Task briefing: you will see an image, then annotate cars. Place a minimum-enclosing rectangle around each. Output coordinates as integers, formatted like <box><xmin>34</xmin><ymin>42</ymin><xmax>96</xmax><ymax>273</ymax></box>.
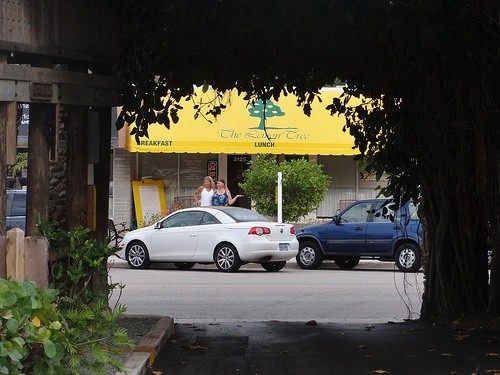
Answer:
<box><xmin>7</xmin><ymin>186</ymin><xmax>27</xmax><ymax>234</ymax></box>
<box><xmin>124</xmin><ymin>206</ymin><xmax>299</xmax><ymax>273</ymax></box>
<box><xmin>296</xmin><ymin>200</ymin><xmax>424</xmax><ymax>273</ymax></box>
